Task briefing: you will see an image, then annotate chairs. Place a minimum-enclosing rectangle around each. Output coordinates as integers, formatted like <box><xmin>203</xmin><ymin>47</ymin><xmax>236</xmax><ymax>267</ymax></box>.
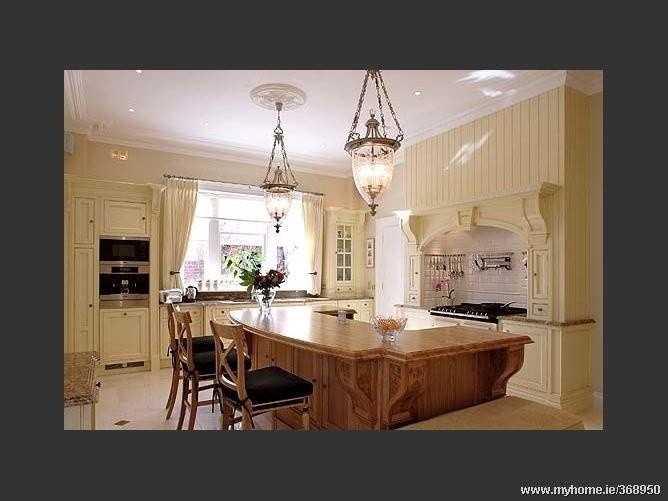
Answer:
<box><xmin>159</xmin><ymin>303</ymin><xmax>312</xmax><ymax>431</ymax></box>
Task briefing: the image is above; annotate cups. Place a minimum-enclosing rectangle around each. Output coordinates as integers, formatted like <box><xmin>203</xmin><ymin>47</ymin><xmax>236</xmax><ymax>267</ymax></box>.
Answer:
<box><xmin>338</xmin><ymin>311</ymin><xmax>346</xmax><ymax>323</ymax></box>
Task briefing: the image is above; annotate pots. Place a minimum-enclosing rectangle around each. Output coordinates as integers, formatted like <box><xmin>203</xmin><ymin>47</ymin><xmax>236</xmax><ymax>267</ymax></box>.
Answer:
<box><xmin>480</xmin><ymin>301</ymin><xmax>515</xmax><ymax>310</ymax></box>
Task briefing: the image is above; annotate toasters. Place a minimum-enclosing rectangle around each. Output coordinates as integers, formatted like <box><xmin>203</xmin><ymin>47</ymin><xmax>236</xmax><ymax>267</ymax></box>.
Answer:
<box><xmin>159</xmin><ymin>290</ymin><xmax>182</xmax><ymax>303</ymax></box>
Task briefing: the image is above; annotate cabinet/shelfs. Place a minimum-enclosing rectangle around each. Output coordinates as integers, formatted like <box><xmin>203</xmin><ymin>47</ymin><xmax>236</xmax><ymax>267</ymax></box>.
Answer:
<box><xmin>101</xmin><ymin>198</ymin><xmax>152</xmax><ymax>236</ymax></box>
<box><xmin>162</xmin><ymin>304</ymin><xmax>235</xmax><ymax>368</ymax></box>
<box><xmin>65</xmin><ymin>173</ymin><xmax>100</xmax><ymax>376</ymax></box>
<box><xmin>100</xmin><ymin>308</ymin><xmax>150</xmax><ymax>370</ymax></box>
<box><xmin>226</xmin><ymin>330</ymin><xmax>351</xmax><ymax>430</ymax></box>
<box><xmin>338</xmin><ymin>300</ymin><xmax>375</xmax><ymax>321</ymax></box>
<box><xmin>322</xmin><ymin>208</ymin><xmax>365</xmax><ymax>298</ymax></box>
<box><xmin>401</xmin><ymin>311</ymin><xmax>551</xmax><ymax>396</ymax></box>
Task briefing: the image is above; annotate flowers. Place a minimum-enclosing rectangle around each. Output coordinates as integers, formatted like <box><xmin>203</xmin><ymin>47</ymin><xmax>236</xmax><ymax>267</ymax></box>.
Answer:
<box><xmin>223</xmin><ymin>245</ymin><xmax>288</xmax><ymax>307</ymax></box>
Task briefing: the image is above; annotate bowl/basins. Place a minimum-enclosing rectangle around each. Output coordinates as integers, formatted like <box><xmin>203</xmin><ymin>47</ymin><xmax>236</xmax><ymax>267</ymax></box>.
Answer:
<box><xmin>370</xmin><ymin>316</ymin><xmax>409</xmax><ymax>343</ymax></box>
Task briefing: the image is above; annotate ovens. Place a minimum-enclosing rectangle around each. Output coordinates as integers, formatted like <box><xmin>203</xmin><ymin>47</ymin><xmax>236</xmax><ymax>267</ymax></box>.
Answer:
<box><xmin>99</xmin><ymin>265</ymin><xmax>151</xmax><ymax>364</ymax></box>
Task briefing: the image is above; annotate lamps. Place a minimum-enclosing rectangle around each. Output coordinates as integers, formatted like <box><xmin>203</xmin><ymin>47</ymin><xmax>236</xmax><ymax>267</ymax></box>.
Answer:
<box><xmin>249</xmin><ymin>83</ymin><xmax>310</xmax><ymax>236</ymax></box>
<box><xmin>343</xmin><ymin>69</ymin><xmax>405</xmax><ymax>218</ymax></box>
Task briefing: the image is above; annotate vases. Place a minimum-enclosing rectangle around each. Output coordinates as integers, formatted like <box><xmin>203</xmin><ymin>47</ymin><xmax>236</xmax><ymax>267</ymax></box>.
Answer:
<box><xmin>252</xmin><ymin>287</ymin><xmax>277</xmax><ymax>308</ymax></box>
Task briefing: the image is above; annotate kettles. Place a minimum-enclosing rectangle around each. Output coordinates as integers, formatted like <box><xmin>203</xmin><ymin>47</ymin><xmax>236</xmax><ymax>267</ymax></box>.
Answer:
<box><xmin>186</xmin><ymin>286</ymin><xmax>198</xmax><ymax>300</ymax></box>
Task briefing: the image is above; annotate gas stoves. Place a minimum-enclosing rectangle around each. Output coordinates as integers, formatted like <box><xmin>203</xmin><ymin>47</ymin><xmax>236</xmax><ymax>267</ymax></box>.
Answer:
<box><xmin>429</xmin><ymin>303</ymin><xmax>526</xmax><ymax>323</ymax></box>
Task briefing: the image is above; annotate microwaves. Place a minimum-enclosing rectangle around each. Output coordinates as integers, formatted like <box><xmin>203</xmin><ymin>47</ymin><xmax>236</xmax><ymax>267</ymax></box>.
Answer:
<box><xmin>99</xmin><ymin>235</ymin><xmax>150</xmax><ymax>267</ymax></box>
